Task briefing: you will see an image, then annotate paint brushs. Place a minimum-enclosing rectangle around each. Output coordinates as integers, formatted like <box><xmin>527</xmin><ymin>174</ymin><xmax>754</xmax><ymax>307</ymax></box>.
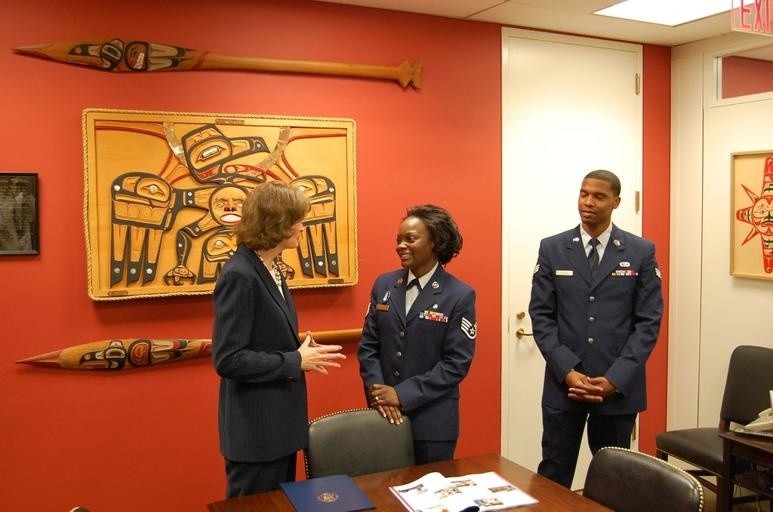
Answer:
<box><xmin>10</xmin><ymin>37</ymin><xmax>424</xmax><ymax>90</ymax></box>
<box><xmin>15</xmin><ymin>325</ymin><xmax>369</xmax><ymax>375</ymax></box>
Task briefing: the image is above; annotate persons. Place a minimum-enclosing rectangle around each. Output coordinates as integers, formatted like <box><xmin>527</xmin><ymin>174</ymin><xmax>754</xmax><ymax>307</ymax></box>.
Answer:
<box><xmin>356</xmin><ymin>202</ymin><xmax>476</xmax><ymax>469</ymax></box>
<box><xmin>212</xmin><ymin>181</ymin><xmax>346</xmax><ymax>502</ymax></box>
<box><xmin>528</xmin><ymin>169</ymin><xmax>664</xmax><ymax>492</ymax></box>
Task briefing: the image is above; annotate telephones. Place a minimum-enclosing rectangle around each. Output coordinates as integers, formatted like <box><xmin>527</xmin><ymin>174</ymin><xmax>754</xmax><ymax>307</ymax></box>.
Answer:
<box><xmin>743</xmin><ymin>405</ymin><xmax>773</xmax><ymax>433</ymax></box>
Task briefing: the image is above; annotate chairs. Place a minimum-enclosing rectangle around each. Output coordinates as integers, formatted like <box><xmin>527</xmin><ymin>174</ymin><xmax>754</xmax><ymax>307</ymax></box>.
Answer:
<box><xmin>581</xmin><ymin>442</ymin><xmax>706</xmax><ymax>512</ymax></box>
<box><xmin>654</xmin><ymin>343</ymin><xmax>772</xmax><ymax>512</ymax></box>
<box><xmin>303</xmin><ymin>405</ymin><xmax>417</xmax><ymax>482</ymax></box>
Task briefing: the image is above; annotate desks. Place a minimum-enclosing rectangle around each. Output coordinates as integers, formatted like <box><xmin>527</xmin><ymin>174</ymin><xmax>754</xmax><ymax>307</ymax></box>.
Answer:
<box><xmin>713</xmin><ymin>429</ymin><xmax>772</xmax><ymax>512</ymax></box>
<box><xmin>205</xmin><ymin>446</ymin><xmax>619</xmax><ymax>511</ymax></box>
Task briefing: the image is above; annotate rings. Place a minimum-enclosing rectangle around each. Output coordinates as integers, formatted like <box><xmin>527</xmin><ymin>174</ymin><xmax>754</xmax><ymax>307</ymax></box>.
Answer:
<box><xmin>375</xmin><ymin>396</ymin><xmax>380</xmax><ymax>401</ymax></box>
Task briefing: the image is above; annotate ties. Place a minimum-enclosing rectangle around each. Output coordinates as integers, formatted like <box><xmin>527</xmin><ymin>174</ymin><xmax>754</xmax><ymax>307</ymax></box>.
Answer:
<box><xmin>587</xmin><ymin>238</ymin><xmax>601</xmax><ymax>282</ymax></box>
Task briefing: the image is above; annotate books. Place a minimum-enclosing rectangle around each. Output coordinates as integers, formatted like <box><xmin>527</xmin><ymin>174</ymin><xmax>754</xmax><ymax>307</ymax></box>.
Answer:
<box><xmin>389</xmin><ymin>470</ymin><xmax>540</xmax><ymax>512</ymax></box>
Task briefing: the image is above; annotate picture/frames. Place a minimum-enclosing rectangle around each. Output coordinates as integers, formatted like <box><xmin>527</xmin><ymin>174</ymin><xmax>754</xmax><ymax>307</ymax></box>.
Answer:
<box><xmin>0</xmin><ymin>170</ymin><xmax>40</xmax><ymax>258</ymax></box>
<box><xmin>727</xmin><ymin>149</ymin><xmax>772</xmax><ymax>284</ymax></box>
<box><xmin>77</xmin><ymin>103</ymin><xmax>360</xmax><ymax>304</ymax></box>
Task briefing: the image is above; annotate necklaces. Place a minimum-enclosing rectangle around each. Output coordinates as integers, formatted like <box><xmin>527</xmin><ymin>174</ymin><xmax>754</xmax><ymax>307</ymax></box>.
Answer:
<box><xmin>258</xmin><ymin>250</ymin><xmax>282</xmax><ymax>286</ymax></box>
